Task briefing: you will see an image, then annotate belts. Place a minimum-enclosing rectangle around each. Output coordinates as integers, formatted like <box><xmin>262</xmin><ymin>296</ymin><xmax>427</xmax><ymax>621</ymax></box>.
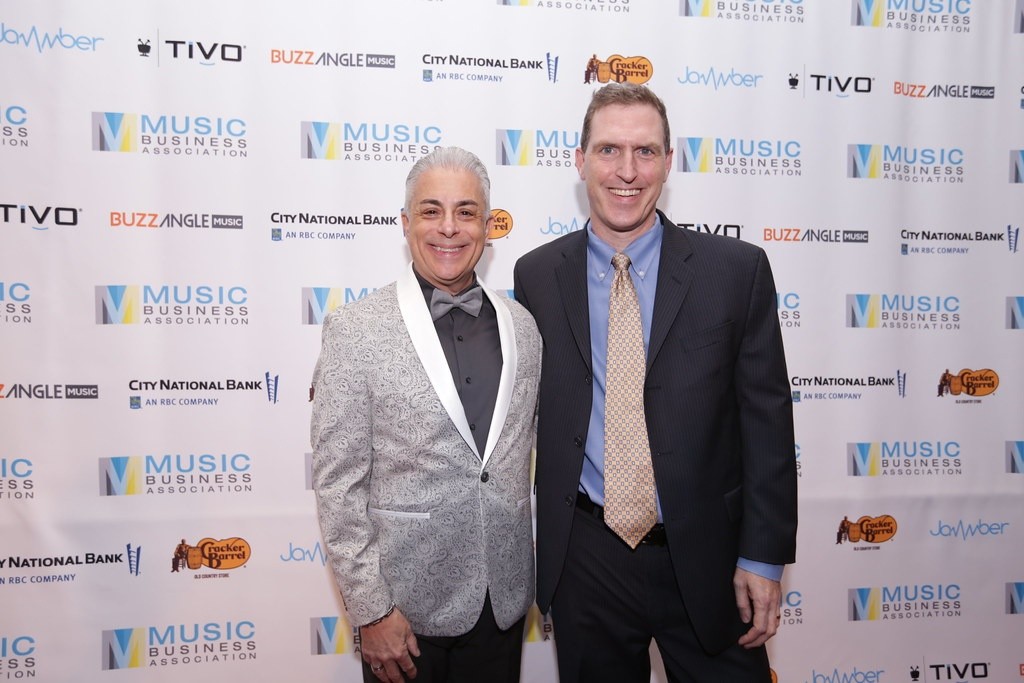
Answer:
<box><xmin>573</xmin><ymin>492</ymin><xmax>667</xmax><ymax>547</ymax></box>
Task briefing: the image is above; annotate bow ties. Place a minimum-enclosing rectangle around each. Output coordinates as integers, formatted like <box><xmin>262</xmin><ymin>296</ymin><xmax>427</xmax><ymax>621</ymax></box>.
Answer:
<box><xmin>430</xmin><ymin>286</ymin><xmax>483</xmax><ymax>322</ymax></box>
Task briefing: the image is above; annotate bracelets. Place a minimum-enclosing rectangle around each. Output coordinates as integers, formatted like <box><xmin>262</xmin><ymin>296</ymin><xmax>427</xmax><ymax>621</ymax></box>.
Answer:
<box><xmin>362</xmin><ymin>608</ymin><xmax>394</xmax><ymax>628</ymax></box>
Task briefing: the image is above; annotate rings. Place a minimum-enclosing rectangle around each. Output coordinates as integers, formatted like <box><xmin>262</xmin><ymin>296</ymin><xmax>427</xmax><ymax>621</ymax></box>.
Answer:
<box><xmin>371</xmin><ymin>664</ymin><xmax>384</xmax><ymax>672</ymax></box>
<box><xmin>777</xmin><ymin>616</ymin><xmax>781</xmax><ymax>619</ymax></box>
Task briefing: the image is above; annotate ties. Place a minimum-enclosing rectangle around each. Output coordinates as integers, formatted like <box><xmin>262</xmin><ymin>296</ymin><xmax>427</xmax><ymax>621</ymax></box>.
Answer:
<box><xmin>603</xmin><ymin>252</ymin><xmax>660</xmax><ymax>551</ymax></box>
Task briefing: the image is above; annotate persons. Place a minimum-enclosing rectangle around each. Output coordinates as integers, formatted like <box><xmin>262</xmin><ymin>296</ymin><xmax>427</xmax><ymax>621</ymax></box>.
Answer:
<box><xmin>311</xmin><ymin>146</ymin><xmax>543</xmax><ymax>683</ymax></box>
<box><xmin>513</xmin><ymin>83</ymin><xmax>799</xmax><ymax>683</ymax></box>
